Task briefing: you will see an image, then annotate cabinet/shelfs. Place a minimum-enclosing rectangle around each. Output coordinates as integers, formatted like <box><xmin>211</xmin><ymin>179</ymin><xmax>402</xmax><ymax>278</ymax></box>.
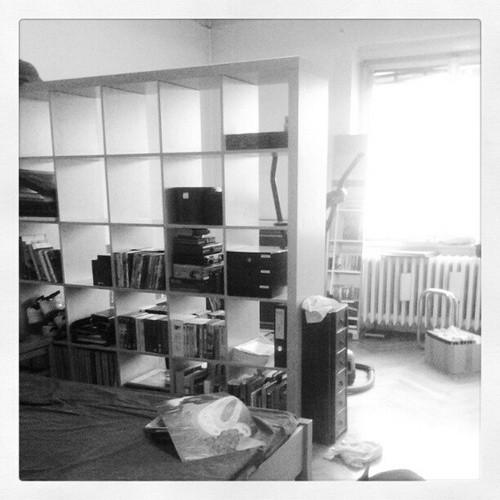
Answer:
<box><xmin>20</xmin><ymin>58</ymin><xmax>327</xmax><ymax>416</ymax></box>
<box><xmin>326</xmin><ymin>133</ymin><xmax>377</xmax><ymax>340</ymax></box>
<box><xmin>302</xmin><ymin>302</ymin><xmax>348</xmax><ymax>446</ymax></box>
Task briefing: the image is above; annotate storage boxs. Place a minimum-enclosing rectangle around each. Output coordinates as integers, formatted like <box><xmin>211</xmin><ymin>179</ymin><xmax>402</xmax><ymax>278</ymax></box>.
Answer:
<box><xmin>423</xmin><ymin>326</ymin><xmax>480</xmax><ymax>376</ymax></box>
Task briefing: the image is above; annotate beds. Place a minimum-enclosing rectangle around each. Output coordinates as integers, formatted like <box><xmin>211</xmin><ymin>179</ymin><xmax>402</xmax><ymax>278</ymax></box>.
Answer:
<box><xmin>19</xmin><ymin>371</ymin><xmax>314</xmax><ymax>481</ymax></box>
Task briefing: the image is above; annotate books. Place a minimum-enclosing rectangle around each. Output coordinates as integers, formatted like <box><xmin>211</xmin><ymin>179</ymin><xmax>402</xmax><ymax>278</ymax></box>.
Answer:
<box><xmin>329</xmin><ymin>212</ymin><xmax>361</xmax><ymax>240</ymax></box>
<box><xmin>325</xmin><ymin>280</ymin><xmax>358</xmax><ymax>302</ymax></box>
<box><xmin>327</xmin><ymin>246</ymin><xmax>362</xmax><ymax>271</ymax></box>
<box><xmin>20</xmin><ymin>232</ymin><xmax>289</xmax><ymax>409</ymax></box>
<box><xmin>19</xmin><ymin>167</ymin><xmax>59</xmax><ymax>218</ymax></box>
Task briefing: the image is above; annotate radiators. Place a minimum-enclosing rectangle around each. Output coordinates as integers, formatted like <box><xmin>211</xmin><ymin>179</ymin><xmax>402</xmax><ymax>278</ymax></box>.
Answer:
<box><xmin>362</xmin><ymin>252</ymin><xmax>480</xmax><ymax>340</ymax></box>
